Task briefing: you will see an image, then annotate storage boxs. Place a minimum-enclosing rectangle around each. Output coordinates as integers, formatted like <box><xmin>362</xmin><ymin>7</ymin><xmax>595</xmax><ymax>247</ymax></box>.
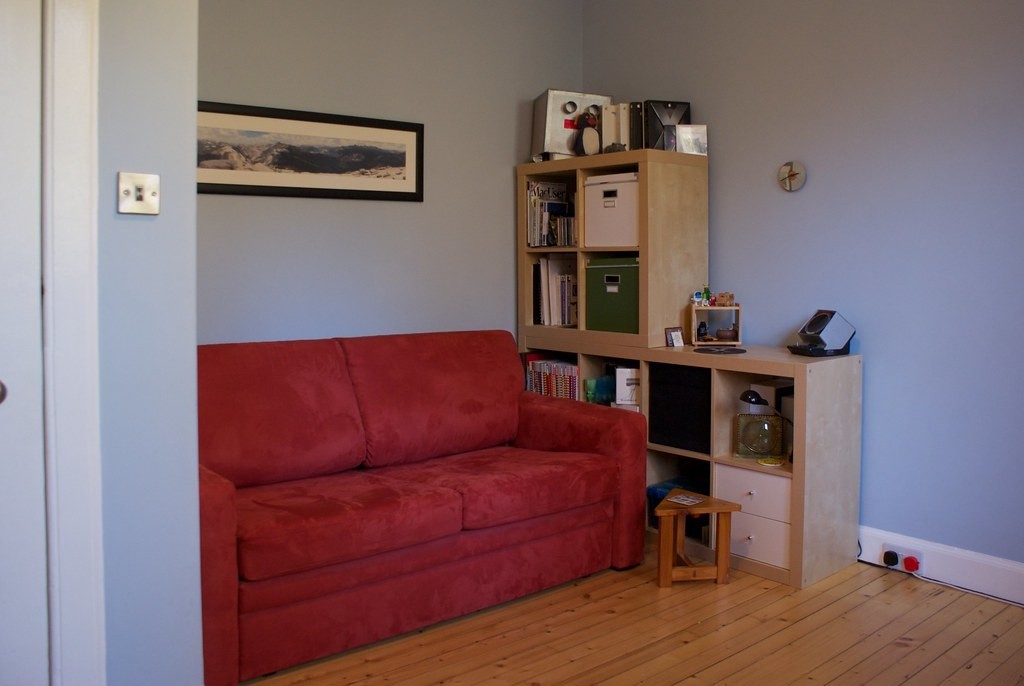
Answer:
<box><xmin>610</xmin><ymin>402</ymin><xmax>640</xmax><ymax>412</ymax></box>
<box><xmin>780</xmin><ymin>395</ymin><xmax>794</xmax><ymax>420</ymax></box>
<box><xmin>584</xmin><ymin>172</ymin><xmax>639</xmax><ymax>247</ymax></box>
<box><xmin>615</xmin><ymin>367</ymin><xmax>642</xmax><ymax>405</ymax></box>
<box><xmin>585</xmin><ymin>259</ymin><xmax>639</xmax><ymax>334</ymax></box>
<box><xmin>750</xmin><ymin>378</ymin><xmax>795</xmax><ymax>411</ymax></box>
<box><xmin>531</xmin><ymin>89</ymin><xmax>707</xmax><ymax>162</ymax></box>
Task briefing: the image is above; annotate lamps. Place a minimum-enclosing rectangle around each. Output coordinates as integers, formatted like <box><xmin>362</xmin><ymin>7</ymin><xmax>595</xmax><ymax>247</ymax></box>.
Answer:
<box><xmin>741</xmin><ymin>389</ymin><xmax>794</xmax><ymax>463</ymax></box>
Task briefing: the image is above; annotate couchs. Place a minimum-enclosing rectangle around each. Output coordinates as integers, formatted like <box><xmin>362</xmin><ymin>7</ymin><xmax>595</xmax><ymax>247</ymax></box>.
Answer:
<box><xmin>198</xmin><ymin>329</ymin><xmax>648</xmax><ymax>686</ymax></box>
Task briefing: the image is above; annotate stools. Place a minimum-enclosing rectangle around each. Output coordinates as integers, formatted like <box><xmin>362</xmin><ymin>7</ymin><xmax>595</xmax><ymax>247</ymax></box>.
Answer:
<box><xmin>655</xmin><ymin>489</ymin><xmax>742</xmax><ymax>589</ymax></box>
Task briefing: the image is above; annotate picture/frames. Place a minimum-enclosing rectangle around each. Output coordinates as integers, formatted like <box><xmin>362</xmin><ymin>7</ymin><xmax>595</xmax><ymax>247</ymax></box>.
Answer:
<box><xmin>197</xmin><ymin>100</ymin><xmax>424</xmax><ymax>202</ymax></box>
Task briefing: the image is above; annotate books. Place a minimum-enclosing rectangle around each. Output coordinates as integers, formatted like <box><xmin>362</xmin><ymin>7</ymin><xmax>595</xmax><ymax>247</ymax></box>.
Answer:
<box><xmin>526</xmin><ymin>178</ymin><xmax>578</xmax><ymax>247</ymax></box>
<box><xmin>531</xmin><ymin>252</ymin><xmax>578</xmax><ymax>329</ymax></box>
<box><xmin>520</xmin><ymin>351</ymin><xmax>578</xmax><ymax>401</ymax></box>
<box><xmin>615</xmin><ymin>367</ymin><xmax>640</xmax><ymax>405</ymax></box>
<box><xmin>583</xmin><ymin>363</ymin><xmax>624</xmax><ymax>407</ymax></box>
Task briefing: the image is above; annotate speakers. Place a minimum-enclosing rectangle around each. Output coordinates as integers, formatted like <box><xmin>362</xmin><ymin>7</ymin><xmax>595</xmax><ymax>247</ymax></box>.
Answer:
<box><xmin>798</xmin><ymin>310</ymin><xmax>855</xmax><ymax>350</ymax></box>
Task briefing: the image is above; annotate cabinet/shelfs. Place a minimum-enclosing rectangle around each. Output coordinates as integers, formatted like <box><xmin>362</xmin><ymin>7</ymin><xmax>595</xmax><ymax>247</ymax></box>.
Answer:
<box><xmin>517</xmin><ymin>147</ymin><xmax>863</xmax><ymax>590</ymax></box>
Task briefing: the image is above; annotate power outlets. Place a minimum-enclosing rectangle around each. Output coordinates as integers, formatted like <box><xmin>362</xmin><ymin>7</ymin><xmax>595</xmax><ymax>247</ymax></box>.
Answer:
<box><xmin>883</xmin><ymin>543</ymin><xmax>924</xmax><ymax>576</ymax></box>
